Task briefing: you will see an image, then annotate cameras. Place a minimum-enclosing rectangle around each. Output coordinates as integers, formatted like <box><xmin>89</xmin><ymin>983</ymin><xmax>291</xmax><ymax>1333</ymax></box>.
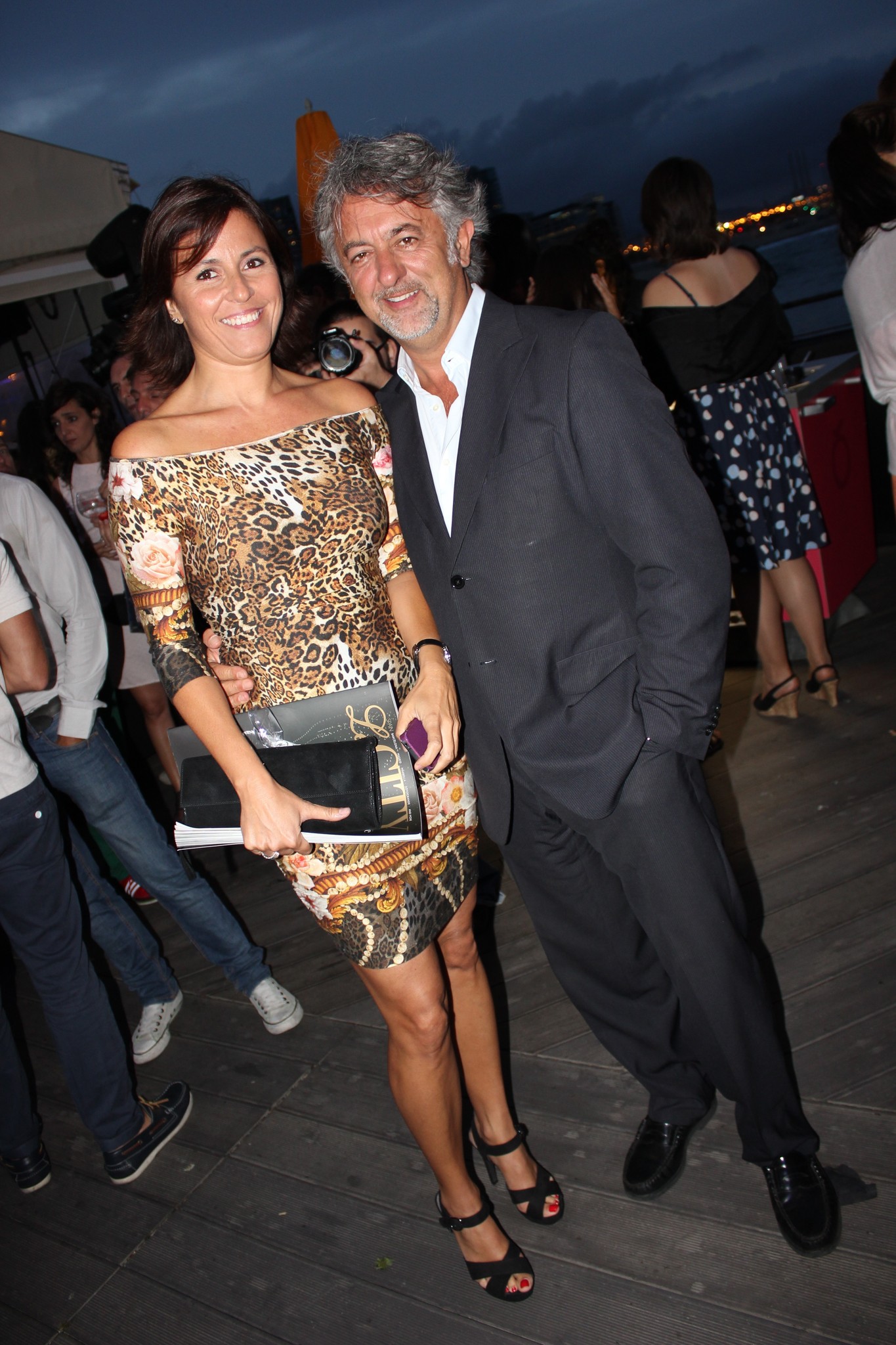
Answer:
<box><xmin>313</xmin><ymin>325</ymin><xmax>365</xmax><ymax>375</ymax></box>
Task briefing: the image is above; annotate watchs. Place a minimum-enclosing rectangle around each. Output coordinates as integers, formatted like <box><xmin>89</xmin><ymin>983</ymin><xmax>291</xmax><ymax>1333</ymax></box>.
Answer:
<box><xmin>411</xmin><ymin>638</ymin><xmax>452</xmax><ymax>674</ymax></box>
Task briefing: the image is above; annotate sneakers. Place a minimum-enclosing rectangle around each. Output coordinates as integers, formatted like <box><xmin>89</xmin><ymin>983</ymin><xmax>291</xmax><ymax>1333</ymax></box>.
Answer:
<box><xmin>119</xmin><ymin>877</ymin><xmax>159</xmax><ymax>906</ymax></box>
<box><xmin>249</xmin><ymin>976</ymin><xmax>303</xmax><ymax>1035</ymax></box>
<box><xmin>129</xmin><ymin>988</ymin><xmax>183</xmax><ymax>1065</ymax></box>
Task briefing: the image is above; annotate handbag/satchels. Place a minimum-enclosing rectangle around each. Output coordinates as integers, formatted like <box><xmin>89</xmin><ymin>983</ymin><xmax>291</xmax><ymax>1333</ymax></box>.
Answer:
<box><xmin>181</xmin><ymin>735</ymin><xmax>384</xmax><ymax>834</ymax></box>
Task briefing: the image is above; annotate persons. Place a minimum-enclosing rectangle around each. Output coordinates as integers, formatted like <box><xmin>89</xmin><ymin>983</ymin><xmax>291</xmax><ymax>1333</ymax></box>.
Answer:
<box><xmin>112</xmin><ymin>172</ymin><xmax>568</xmax><ymax>1306</ymax></box>
<box><xmin>826</xmin><ymin>106</ymin><xmax>895</xmax><ymax>518</ymax></box>
<box><xmin>197</xmin><ymin>132</ymin><xmax>841</xmax><ymax>1260</ymax></box>
<box><xmin>0</xmin><ymin>473</ymin><xmax>307</xmax><ymax>1066</ymax></box>
<box><xmin>111</xmin><ymin>347</ymin><xmax>142</xmax><ymax>422</ymax></box>
<box><xmin>125</xmin><ymin>363</ymin><xmax>184</xmax><ymax>420</ymax></box>
<box><xmin>2</xmin><ymin>450</ymin><xmax>163</xmax><ymax>907</ymax></box>
<box><xmin>636</xmin><ymin>156</ymin><xmax>837</xmax><ymax>719</ymax></box>
<box><xmin>0</xmin><ymin>539</ymin><xmax>196</xmax><ymax>1193</ymax></box>
<box><xmin>41</xmin><ymin>378</ymin><xmax>194</xmax><ymax>814</ymax></box>
<box><xmin>487</xmin><ymin>210</ymin><xmax>638</xmax><ymax>345</ymax></box>
<box><xmin>304</xmin><ymin>297</ymin><xmax>397</xmax><ymax>398</ymax></box>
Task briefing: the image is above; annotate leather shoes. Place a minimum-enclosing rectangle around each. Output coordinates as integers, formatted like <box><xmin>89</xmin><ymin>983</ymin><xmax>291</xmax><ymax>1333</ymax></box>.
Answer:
<box><xmin>752</xmin><ymin>1145</ymin><xmax>842</xmax><ymax>1258</ymax></box>
<box><xmin>0</xmin><ymin>1141</ymin><xmax>53</xmax><ymax>1195</ymax></box>
<box><xmin>103</xmin><ymin>1080</ymin><xmax>194</xmax><ymax>1185</ymax></box>
<box><xmin>622</xmin><ymin>1088</ymin><xmax>716</xmax><ymax>1199</ymax></box>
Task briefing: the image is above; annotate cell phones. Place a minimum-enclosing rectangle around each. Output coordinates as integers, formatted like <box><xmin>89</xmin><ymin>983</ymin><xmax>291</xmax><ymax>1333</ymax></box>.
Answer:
<box><xmin>400</xmin><ymin>717</ymin><xmax>442</xmax><ymax>772</ymax></box>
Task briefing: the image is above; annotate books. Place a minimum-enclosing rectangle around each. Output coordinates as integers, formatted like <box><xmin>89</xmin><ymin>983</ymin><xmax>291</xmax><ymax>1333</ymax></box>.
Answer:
<box><xmin>162</xmin><ymin>680</ymin><xmax>427</xmax><ymax>850</ymax></box>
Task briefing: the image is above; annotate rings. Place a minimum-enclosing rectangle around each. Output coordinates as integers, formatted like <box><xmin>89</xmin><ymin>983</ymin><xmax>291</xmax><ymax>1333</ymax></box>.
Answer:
<box><xmin>260</xmin><ymin>851</ymin><xmax>281</xmax><ymax>861</ymax></box>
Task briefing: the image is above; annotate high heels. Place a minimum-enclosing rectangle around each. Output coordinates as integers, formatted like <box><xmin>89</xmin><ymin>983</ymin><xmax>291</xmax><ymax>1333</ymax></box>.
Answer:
<box><xmin>805</xmin><ymin>663</ymin><xmax>841</xmax><ymax>706</ymax></box>
<box><xmin>436</xmin><ymin>1175</ymin><xmax>536</xmax><ymax>1302</ymax></box>
<box><xmin>753</xmin><ymin>674</ymin><xmax>801</xmax><ymax>720</ymax></box>
<box><xmin>469</xmin><ymin>1120</ymin><xmax>566</xmax><ymax>1225</ymax></box>
